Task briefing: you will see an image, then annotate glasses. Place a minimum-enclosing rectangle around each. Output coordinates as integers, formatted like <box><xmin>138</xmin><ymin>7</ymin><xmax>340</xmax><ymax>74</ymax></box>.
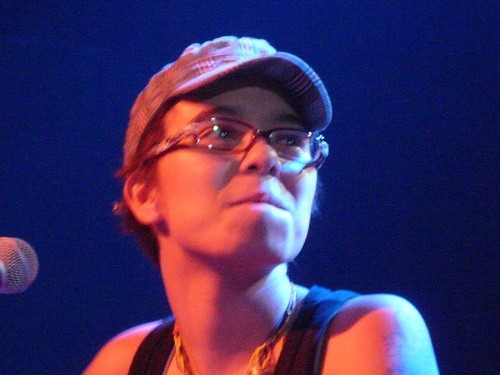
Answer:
<box><xmin>137</xmin><ymin>117</ymin><xmax>329</xmax><ymax>170</ymax></box>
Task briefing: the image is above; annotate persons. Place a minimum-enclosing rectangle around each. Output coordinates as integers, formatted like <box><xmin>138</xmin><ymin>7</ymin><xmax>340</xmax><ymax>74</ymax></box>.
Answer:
<box><xmin>82</xmin><ymin>35</ymin><xmax>441</xmax><ymax>375</ymax></box>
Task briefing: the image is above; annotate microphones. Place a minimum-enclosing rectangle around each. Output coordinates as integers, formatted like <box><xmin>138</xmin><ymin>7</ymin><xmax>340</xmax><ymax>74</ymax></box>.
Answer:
<box><xmin>0</xmin><ymin>237</ymin><xmax>39</xmax><ymax>295</ymax></box>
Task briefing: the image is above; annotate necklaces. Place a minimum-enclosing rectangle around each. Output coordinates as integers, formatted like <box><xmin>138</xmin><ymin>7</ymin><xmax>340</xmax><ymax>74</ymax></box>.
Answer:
<box><xmin>173</xmin><ymin>282</ymin><xmax>297</xmax><ymax>375</ymax></box>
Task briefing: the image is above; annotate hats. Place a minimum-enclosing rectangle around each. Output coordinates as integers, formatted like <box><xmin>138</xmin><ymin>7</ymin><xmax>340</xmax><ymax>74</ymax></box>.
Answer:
<box><xmin>123</xmin><ymin>36</ymin><xmax>332</xmax><ymax>175</ymax></box>
